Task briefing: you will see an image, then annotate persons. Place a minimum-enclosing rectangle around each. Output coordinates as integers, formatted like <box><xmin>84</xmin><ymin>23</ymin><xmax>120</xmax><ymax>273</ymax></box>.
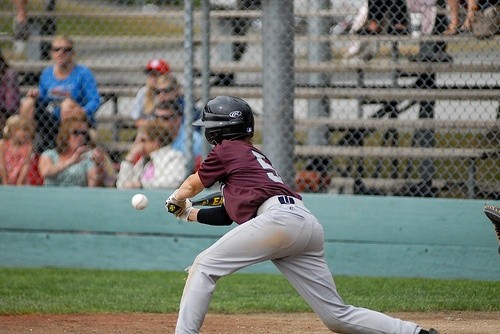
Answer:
<box><xmin>0</xmin><ymin>1</ymin><xmax>216</xmax><ymax>189</ymax></box>
<box><xmin>356</xmin><ymin>0</ymin><xmax>500</xmax><ymax>38</ymax></box>
<box><xmin>163</xmin><ymin>95</ymin><xmax>441</xmax><ymax>334</ymax></box>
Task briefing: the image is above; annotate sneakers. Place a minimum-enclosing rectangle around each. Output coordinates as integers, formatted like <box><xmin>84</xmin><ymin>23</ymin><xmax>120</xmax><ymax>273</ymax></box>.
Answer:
<box><xmin>484</xmin><ymin>205</ymin><xmax>500</xmax><ymax>240</ymax></box>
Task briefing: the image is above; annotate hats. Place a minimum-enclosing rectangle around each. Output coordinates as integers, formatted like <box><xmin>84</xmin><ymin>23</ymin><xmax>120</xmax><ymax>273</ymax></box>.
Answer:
<box><xmin>143</xmin><ymin>58</ymin><xmax>169</xmax><ymax>74</ymax></box>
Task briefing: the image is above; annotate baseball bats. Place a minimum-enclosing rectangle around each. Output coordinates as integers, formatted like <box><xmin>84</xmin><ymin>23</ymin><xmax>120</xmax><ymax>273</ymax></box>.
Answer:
<box><xmin>168</xmin><ymin>191</ymin><xmax>223</xmax><ymax>213</ymax></box>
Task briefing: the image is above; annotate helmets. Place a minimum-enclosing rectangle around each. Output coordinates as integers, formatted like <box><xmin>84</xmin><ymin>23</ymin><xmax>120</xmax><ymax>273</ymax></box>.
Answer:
<box><xmin>192</xmin><ymin>96</ymin><xmax>254</xmax><ymax>145</ymax></box>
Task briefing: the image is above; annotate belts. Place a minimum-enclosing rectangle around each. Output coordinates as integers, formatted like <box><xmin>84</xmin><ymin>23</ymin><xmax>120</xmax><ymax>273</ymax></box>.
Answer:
<box><xmin>278</xmin><ymin>196</ymin><xmax>295</xmax><ymax>204</ymax></box>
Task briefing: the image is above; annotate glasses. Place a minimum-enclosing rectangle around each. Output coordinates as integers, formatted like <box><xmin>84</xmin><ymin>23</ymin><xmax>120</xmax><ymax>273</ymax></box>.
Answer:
<box><xmin>69</xmin><ymin>129</ymin><xmax>88</xmax><ymax>136</ymax></box>
<box><xmin>153</xmin><ymin>113</ymin><xmax>178</xmax><ymax>120</ymax></box>
<box><xmin>51</xmin><ymin>47</ymin><xmax>73</xmax><ymax>52</ymax></box>
<box><xmin>155</xmin><ymin>87</ymin><xmax>174</xmax><ymax>95</ymax></box>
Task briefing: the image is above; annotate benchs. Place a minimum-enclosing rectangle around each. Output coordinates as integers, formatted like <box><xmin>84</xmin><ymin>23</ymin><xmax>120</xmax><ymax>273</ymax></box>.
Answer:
<box><xmin>0</xmin><ymin>0</ymin><xmax>500</xmax><ymax>198</ymax></box>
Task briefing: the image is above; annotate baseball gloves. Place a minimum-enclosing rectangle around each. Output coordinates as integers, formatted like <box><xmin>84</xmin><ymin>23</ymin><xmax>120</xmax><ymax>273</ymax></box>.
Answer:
<box><xmin>484</xmin><ymin>205</ymin><xmax>500</xmax><ymax>254</ymax></box>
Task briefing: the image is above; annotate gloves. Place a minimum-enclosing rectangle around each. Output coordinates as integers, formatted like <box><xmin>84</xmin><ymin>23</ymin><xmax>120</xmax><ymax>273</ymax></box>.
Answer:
<box><xmin>165</xmin><ymin>189</ymin><xmax>193</xmax><ymax>222</ymax></box>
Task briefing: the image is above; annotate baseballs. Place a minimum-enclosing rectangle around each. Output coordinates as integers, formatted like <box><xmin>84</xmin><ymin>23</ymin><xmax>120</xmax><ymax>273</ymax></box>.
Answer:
<box><xmin>132</xmin><ymin>193</ymin><xmax>148</xmax><ymax>211</ymax></box>
